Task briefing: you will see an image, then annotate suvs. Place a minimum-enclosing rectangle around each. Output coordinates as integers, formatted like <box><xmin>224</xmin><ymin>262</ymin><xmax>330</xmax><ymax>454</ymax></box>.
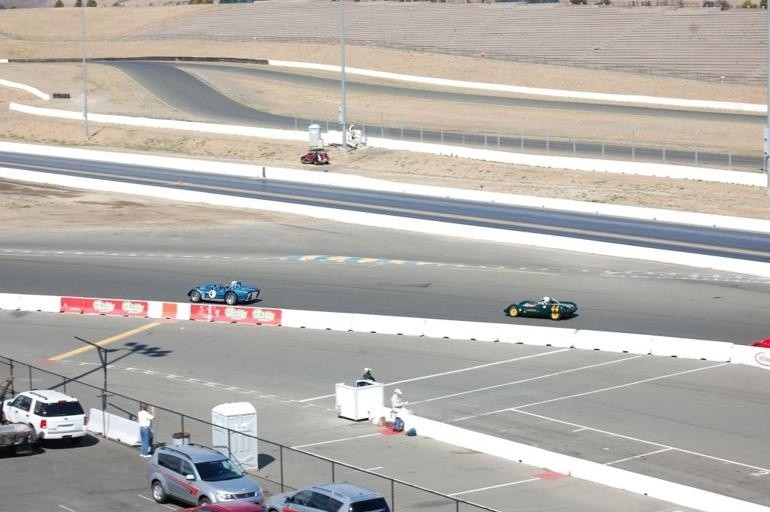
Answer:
<box><xmin>269</xmin><ymin>478</ymin><xmax>392</xmax><ymax>511</ymax></box>
<box><xmin>2</xmin><ymin>387</ymin><xmax>89</xmax><ymax>443</ymax></box>
<box><xmin>146</xmin><ymin>440</ymin><xmax>264</xmax><ymax>508</ymax></box>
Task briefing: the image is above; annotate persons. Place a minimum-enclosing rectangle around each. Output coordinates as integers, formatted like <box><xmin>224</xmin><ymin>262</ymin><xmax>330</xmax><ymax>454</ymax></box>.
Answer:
<box><xmin>541</xmin><ymin>296</ymin><xmax>549</xmax><ymax>303</ymax></box>
<box><xmin>137</xmin><ymin>403</ymin><xmax>156</xmax><ymax>457</ymax></box>
<box><xmin>362</xmin><ymin>369</ymin><xmax>376</xmax><ymax>382</ymax></box>
<box><xmin>391</xmin><ymin>388</ymin><xmax>406</xmax><ymax>410</ymax></box>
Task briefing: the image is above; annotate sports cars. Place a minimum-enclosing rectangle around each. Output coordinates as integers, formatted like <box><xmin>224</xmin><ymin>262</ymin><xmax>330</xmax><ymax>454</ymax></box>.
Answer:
<box><xmin>505</xmin><ymin>294</ymin><xmax>579</xmax><ymax>323</ymax></box>
<box><xmin>186</xmin><ymin>280</ymin><xmax>264</xmax><ymax>304</ymax></box>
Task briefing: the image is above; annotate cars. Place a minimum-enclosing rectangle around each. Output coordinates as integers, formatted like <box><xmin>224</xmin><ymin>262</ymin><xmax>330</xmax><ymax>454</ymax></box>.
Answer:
<box><xmin>172</xmin><ymin>500</ymin><xmax>269</xmax><ymax>511</ymax></box>
<box><xmin>300</xmin><ymin>146</ymin><xmax>331</xmax><ymax>164</ymax></box>
<box><xmin>0</xmin><ymin>412</ymin><xmax>35</xmax><ymax>456</ymax></box>
<box><xmin>751</xmin><ymin>337</ymin><xmax>770</xmax><ymax>350</ymax></box>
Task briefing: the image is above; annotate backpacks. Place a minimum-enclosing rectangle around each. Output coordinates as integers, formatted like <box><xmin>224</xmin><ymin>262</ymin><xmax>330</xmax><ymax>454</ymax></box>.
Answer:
<box><xmin>393</xmin><ymin>416</ymin><xmax>404</xmax><ymax>432</ymax></box>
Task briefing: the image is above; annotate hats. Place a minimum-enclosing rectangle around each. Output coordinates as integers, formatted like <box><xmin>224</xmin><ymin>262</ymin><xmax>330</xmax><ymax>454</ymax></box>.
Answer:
<box><xmin>394</xmin><ymin>389</ymin><xmax>402</xmax><ymax>395</ymax></box>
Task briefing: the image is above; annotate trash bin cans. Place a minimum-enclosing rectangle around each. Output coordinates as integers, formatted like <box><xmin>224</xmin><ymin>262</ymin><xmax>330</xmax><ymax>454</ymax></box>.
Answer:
<box><xmin>173</xmin><ymin>432</ymin><xmax>191</xmax><ymax>447</ymax></box>
